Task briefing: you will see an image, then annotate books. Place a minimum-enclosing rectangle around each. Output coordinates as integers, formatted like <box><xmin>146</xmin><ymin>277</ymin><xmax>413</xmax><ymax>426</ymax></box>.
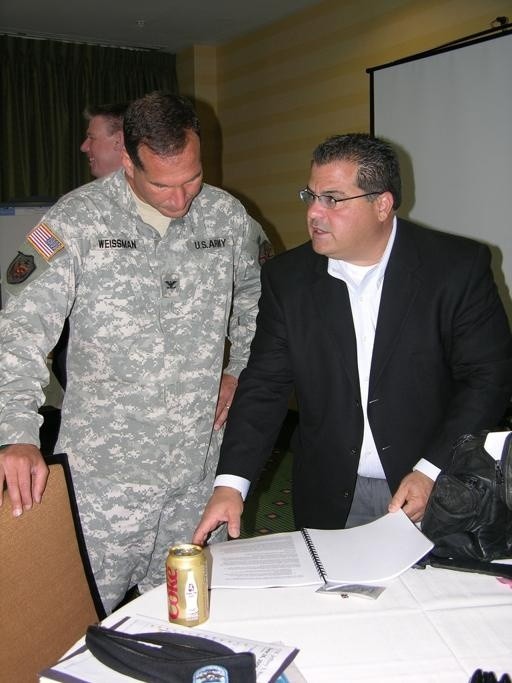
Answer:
<box><xmin>202</xmin><ymin>507</ymin><xmax>435</xmax><ymax>590</ymax></box>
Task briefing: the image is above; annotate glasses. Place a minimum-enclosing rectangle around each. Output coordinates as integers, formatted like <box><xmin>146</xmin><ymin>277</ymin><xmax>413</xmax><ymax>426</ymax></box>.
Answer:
<box><xmin>298</xmin><ymin>187</ymin><xmax>381</xmax><ymax>209</ymax></box>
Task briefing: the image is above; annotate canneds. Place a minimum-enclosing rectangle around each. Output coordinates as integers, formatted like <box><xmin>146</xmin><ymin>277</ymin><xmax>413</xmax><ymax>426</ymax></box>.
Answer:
<box><xmin>165</xmin><ymin>543</ymin><xmax>208</xmax><ymax>625</ymax></box>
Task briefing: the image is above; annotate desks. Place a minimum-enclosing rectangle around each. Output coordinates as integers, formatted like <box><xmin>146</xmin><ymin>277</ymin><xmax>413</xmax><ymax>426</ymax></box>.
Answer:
<box><xmin>36</xmin><ymin>532</ymin><xmax>512</xmax><ymax>680</ymax></box>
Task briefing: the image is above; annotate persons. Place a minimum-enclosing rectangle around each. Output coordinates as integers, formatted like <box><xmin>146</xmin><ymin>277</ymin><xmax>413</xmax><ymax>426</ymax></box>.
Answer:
<box><xmin>191</xmin><ymin>130</ymin><xmax>512</xmax><ymax>570</ymax></box>
<box><xmin>0</xmin><ymin>89</ymin><xmax>264</xmax><ymax>621</ymax></box>
<box><xmin>80</xmin><ymin>101</ymin><xmax>126</xmax><ymax>182</ymax></box>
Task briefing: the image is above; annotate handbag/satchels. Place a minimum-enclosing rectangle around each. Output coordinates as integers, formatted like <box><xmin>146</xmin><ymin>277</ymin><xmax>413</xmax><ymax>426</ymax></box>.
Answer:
<box><xmin>420</xmin><ymin>424</ymin><xmax>512</xmax><ymax>564</ymax></box>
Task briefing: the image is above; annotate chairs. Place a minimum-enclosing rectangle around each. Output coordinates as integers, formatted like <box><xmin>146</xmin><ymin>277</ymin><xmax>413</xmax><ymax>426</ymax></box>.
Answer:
<box><xmin>1</xmin><ymin>454</ymin><xmax>104</xmax><ymax>680</ymax></box>
